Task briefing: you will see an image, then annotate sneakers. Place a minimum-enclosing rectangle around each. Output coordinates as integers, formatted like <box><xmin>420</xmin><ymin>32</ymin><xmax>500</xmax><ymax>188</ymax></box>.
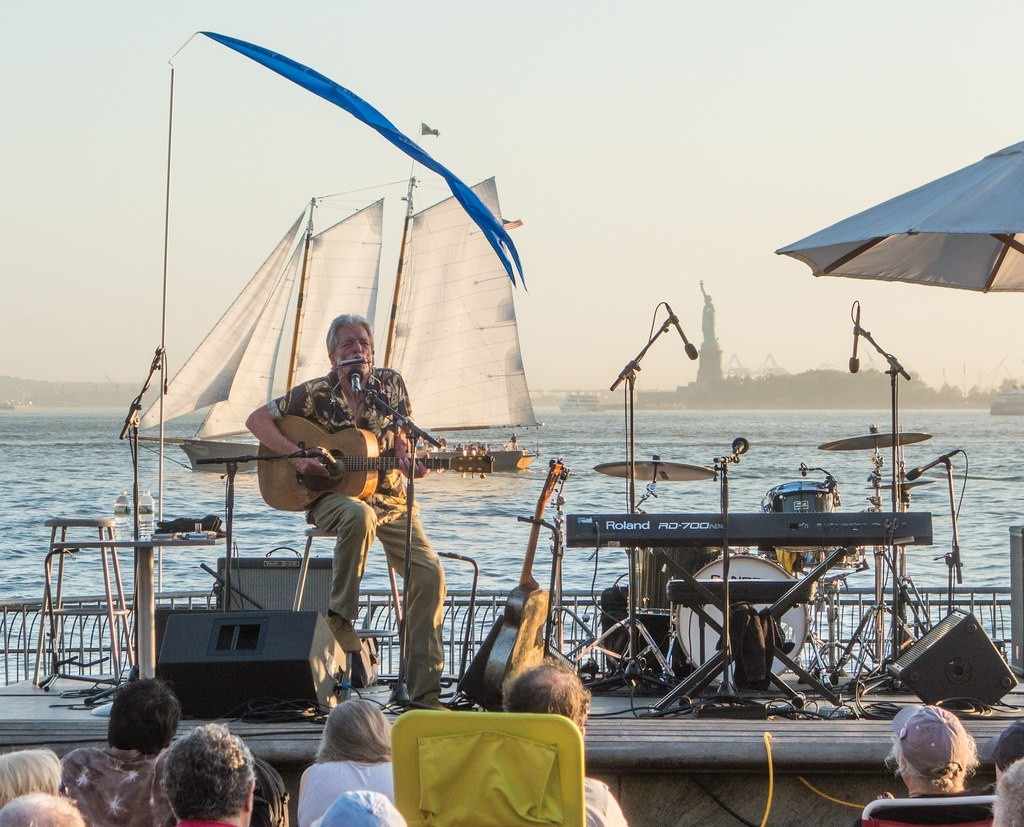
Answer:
<box><xmin>406</xmin><ymin>699</ymin><xmax>451</xmax><ymax>712</ymax></box>
<box><xmin>325</xmin><ymin>613</ymin><xmax>361</xmax><ymax>652</ymax></box>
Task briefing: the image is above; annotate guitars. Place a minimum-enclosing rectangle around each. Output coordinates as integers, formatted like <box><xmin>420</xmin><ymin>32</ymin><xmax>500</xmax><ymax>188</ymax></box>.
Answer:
<box><xmin>257</xmin><ymin>415</ymin><xmax>494</xmax><ymax>513</ymax></box>
<box><xmin>471</xmin><ymin>459</ymin><xmax>570</xmax><ymax>719</ymax></box>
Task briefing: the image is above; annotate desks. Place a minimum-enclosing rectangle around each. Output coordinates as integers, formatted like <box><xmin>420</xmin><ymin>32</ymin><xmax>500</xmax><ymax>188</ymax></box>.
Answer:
<box><xmin>49</xmin><ymin>539</ymin><xmax>222</xmax><ymax>717</ymax></box>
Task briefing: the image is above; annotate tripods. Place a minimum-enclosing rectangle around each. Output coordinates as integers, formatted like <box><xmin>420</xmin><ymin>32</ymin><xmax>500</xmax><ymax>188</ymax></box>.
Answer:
<box><xmin>572</xmin><ymin>320</ymin><xmax>934</xmax><ymax>720</ymax></box>
<box><xmin>350</xmin><ymin>383</ymin><xmax>444</xmax><ymax>711</ymax></box>
<box><xmin>83</xmin><ymin>344</ymin><xmax>165</xmax><ymax>701</ymax></box>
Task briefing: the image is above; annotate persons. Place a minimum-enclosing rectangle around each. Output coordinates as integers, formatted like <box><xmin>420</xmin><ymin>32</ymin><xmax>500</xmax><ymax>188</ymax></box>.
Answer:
<box><xmin>990</xmin><ymin>756</ymin><xmax>1024</xmax><ymax>827</ymax></box>
<box><xmin>509</xmin><ymin>433</ymin><xmax>518</xmax><ymax>451</ymax></box>
<box><xmin>980</xmin><ymin>717</ymin><xmax>1024</xmax><ymax>784</ymax></box>
<box><xmin>305</xmin><ymin>789</ymin><xmax>407</xmax><ymax>827</ymax></box>
<box><xmin>854</xmin><ymin>703</ymin><xmax>997</xmax><ymax>827</ymax></box>
<box><xmin>245</xmin><ymin>313</ymin><xmax>454</xmax><ymax>708</ymax></box>
<box><xmin>1</xmin><ymin>750</ymin><xmax>70</xmax><ymax>809</ymax></box>
<box><xmin>56</xmin><ymin>676</ymin><xmax>179</xmax><ymax>826</ymax></box>
<box><xmin>699</xmin><ymin>280</ymin><xmax>716</xmax><ymax>342</ymax></box>
<box><xmin>0</xmin><ymin>795</ymin><xmax>86</xmax><ymax>827</ymax></box>
<box><xmin>436</xmin><ymin>436</ymin><xmax>491</xmax><ymax>453</ymax></box>
<box><xmin>294</xmin><ymin>698</ymin><xmax>395</xmax><ymax>826</ymax></box>
<box><xmin>504</xmin><ymin>660</ymin><xmax>628</xmax><ymax>826</ymax></box>
<box><xmin>163</xmin><ymin>720</ymin><xmax>256</xmax><ymax>827</ymax></box>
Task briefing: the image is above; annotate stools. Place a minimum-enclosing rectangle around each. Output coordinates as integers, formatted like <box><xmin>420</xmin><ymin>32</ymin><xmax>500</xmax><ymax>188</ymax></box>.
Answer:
<box><xmin>32</xmin><ymin>519</ymin><xmax>137</xmax><ymax>687</ymax></box>
<box><xmin>291</xmin><ymin>527</ymin><xmax>412</xmax><ymax>706</ymax></box>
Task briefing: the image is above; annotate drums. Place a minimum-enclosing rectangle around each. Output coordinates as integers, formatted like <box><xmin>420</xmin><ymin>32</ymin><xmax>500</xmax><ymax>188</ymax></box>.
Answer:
<box><xmin>676</xmin><ymin>555</ymin><xmax>810</xmax><ymax>687</ymax></box>
<box><xmin>759</xmin><ymin>480</ymin><xmax>836</xmax><ymax>555</ymax></box>
<box><xmin>631</xmin><ymin>541</ymin><xmax>726</xmax><ymax>616</ymax></box>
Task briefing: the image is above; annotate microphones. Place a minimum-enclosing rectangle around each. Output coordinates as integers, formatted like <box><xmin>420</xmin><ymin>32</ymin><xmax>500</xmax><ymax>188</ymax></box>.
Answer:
<box><xmin>850</xmin><ymin>306</ymin><xmax>861</xmax><ymax>373</ymax></box>
<box><xmin>732</xmin><ymin>437</ymin><xmax>750</xmax><ymax>459</ymax></box>
<box><xmin>906</xmin><ymin>450</ymin><xmax>960</xmax><ymax>481</ymax></box>
<box><xmin>664</xmin><ymin>303</ymin><xmax>698</xmax><ymax>360</ymax></box>
<box><xmin>346</xmin><ymin>368</ymin><xmax>364</xmax><ymax>394</ymax></box>
<box><xmin>164</xmin><ymin>377</ymin><xmax>168</xmax><ymax>394</ymax></box>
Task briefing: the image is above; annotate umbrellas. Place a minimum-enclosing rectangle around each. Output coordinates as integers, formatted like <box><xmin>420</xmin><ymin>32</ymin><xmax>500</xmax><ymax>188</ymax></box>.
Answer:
<box><xmin>774</xmin><ymin>138</ymin><xmax>1024</xmax><ymax>293</ymax></box>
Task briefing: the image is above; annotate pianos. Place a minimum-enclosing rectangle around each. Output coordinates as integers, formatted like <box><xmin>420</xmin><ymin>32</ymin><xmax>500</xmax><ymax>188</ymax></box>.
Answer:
<box><xmin>565</xmin><ymin>510</ymin><xmax>935</xmax><ymax>546</ymax></box>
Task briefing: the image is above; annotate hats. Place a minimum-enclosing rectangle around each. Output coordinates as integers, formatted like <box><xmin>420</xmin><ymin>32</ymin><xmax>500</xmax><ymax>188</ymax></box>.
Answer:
<box><xmin>980</xmin><ymin>720</ymin><xmax>1023</xmax><ymax>772</ymax></box>
<box><xmin>892</xmin><ymin>704</ymin><xmax>968</xmax><ymax>780</ymax></box>
<box><xmin>313</xmin><ymin>790</ymin><xmax>406</xmax><ymax>826</ymax></box>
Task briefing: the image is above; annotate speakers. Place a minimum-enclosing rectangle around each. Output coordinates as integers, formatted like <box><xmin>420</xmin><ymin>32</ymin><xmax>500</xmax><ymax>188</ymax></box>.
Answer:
<box><xmin>887</xmin><ymin>608</ymin><xmax>1018</xmax><ymax>712</ymax></box>
<box><xmin>153</xmin><ymin>611</ymin><xmax>351</xmax><ymax>719</ymax></box>
<box><xmin>216</xmin><ymin>557</ymin><xmax>336</xmax><ymax>618</ymax></box>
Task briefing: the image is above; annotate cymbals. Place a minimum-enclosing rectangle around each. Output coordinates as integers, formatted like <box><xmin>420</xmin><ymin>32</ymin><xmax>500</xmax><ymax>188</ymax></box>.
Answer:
<box><xmin>593</xmin><ymin>459</ymin><xmax>720</xmax><ymax>482</ymax></box>
<box><xmin>818</xmin><ymin>431</ymin><xmax>931</xmax><ymax>451</ymax></box>
